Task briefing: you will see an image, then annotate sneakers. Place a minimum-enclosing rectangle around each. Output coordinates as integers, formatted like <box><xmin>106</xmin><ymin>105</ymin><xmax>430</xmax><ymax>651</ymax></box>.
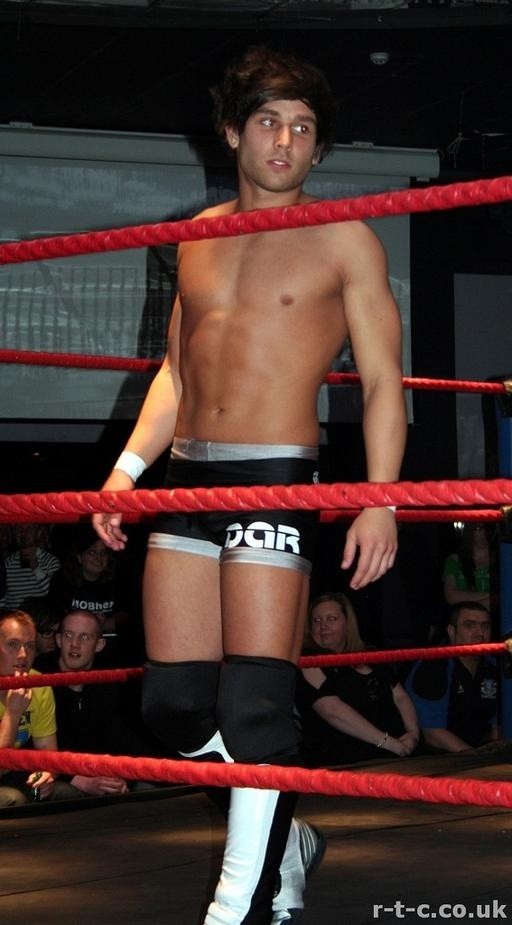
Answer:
<box><xmin>270</xmin><ymin>818</ymin><xmax>327</xmax><ymax>925</ymax></box>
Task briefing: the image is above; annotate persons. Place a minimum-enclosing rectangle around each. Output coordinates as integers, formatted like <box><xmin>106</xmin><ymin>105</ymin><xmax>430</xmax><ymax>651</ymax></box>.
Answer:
<box><xmin>0</xmin><ymin>522</ymin><xmax>511</xmax><ymax>811</ymax></box>
<box><xmin>89</xmin><ymin>54</ymin><xmax>410</xmax><ymax>925</ymax></box>
